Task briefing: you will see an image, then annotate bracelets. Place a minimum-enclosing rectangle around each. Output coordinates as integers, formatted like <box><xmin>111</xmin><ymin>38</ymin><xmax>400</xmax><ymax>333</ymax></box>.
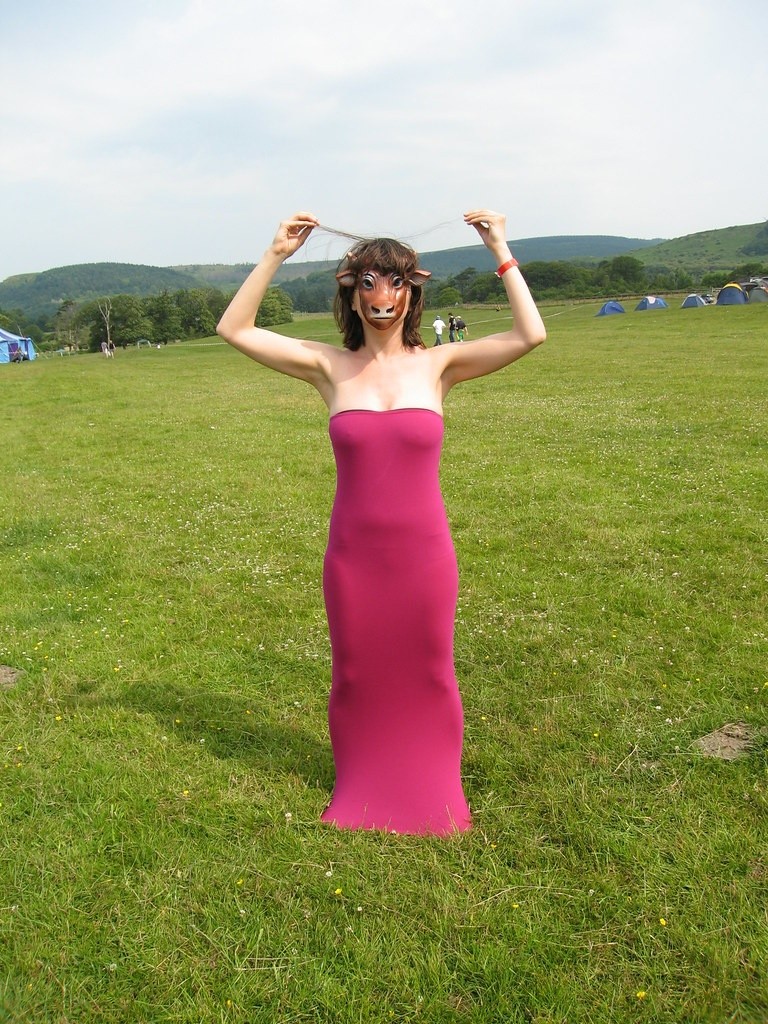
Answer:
<box><xmin>494</xmin><ymin>258</ymin><xmax>520</xmax><ymax>277</ymax></box>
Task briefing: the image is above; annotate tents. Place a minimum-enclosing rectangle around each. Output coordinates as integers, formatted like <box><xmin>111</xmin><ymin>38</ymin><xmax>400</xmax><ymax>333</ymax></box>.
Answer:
<box><xmin>137</xmin><ymin>339</ymin><xmax>151</xmax><ymax>349</ymax></box>
<box><xmin>634</xmin><ymin>297</ymin><xmax>667</xmax><ymax>310</ymax></box>
<box><xmin>682</xmin><ymin>294</ymin><xmax>705</xmax><ymax>308</ymax></box>
<box><xmin>0</xmin><ymin>328</ymin><xmax>36</xmax><ymax>363</ymax></box>
<box><xmin>701</xmin><ymin>280</ymin><xmax>768</xmax><ymax>305</ymax></box>
<box><xmin>594</xmin><ymin>302</ymin><xmax>625</xmax><ymax>316</ymax></box>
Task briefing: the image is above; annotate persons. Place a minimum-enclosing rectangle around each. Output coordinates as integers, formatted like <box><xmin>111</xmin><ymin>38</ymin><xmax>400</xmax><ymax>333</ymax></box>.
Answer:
<box><xmin>455</xmin><ymin>316</ymin><xmax>468</xmax><ymax>342</ymax></box>
<box><xmin>448</xmin><ymin>313</ymin><xmax>457</xmax><ymax>342</ymax></box>
<box><xmin>215</xmin><ymin>210</ymin><xmax>549</xmax><ymax>833</ymax></box>
<box><xmin>433</xmin><ymin>315</ymin><xmax>446</xmax><ymax>346</ymax></box>
<box><xmin>101</xmin><ymin>340</ymin><xmax>115</xmax><ymax>359</ymax></box>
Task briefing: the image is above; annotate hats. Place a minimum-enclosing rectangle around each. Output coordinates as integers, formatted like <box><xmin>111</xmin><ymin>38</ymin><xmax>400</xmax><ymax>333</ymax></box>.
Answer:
<box><xmin>457</xmin><ymin>316</ymin><xmax>461</xmax><ymax>319</ymax></box>
<box><xmin>455</xmin><ymin>317</ymin><xmax>457</xmax><ymax>319</ymax></box>
<box><xmin>451</xmin><ymin>316</ymin><xmax>454</xmax><ymax>319</ymax></box>
<box><xmin>436</xmin><ymin>315</ymin><xmax>440</xmax><ymax>319</ymax></box>
